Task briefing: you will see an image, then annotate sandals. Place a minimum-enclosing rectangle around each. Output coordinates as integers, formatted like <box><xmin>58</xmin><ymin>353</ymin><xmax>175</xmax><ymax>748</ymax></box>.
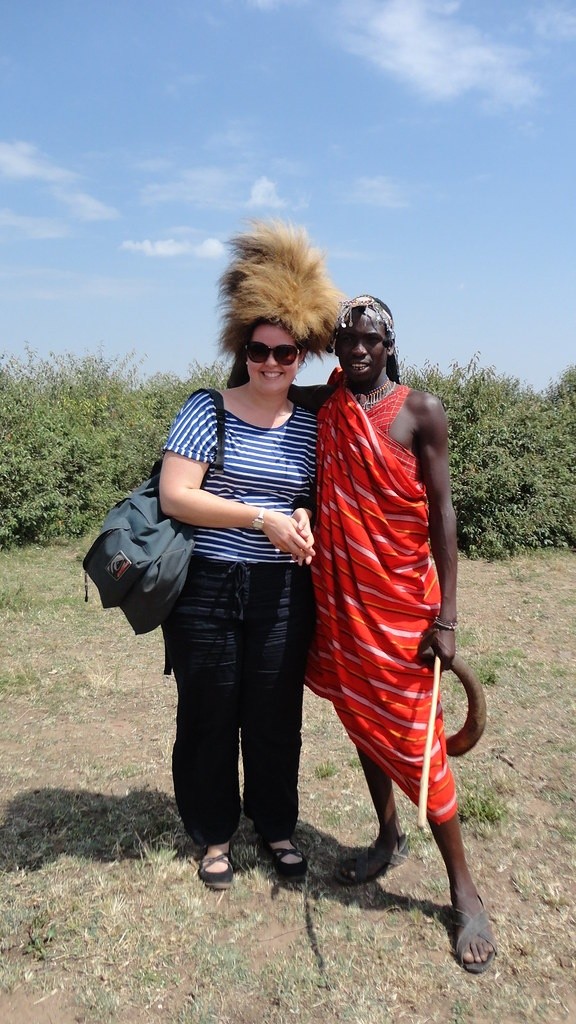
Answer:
<box><xmin>334</xmin><ymin>831</ymin><xmax>411</xmax><ymax>885</ymax></box>
<box><xmin>454</xmin><ymin>895</ymin><xmax>498</xmax><ymax>973</ymax></box>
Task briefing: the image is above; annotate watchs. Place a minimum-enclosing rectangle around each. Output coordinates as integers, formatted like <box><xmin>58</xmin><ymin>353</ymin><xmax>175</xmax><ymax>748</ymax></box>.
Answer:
<box><xmin>252</xmin><ymin>508</ymin><xmax>265</xmax><ymax>530</ymax></box>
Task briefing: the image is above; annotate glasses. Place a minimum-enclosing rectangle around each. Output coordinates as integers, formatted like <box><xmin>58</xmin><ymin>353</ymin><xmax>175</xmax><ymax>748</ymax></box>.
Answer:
<box><xmin>244</xmin><ymin>342</ymin><xmax>301</xmax><ymax>366</ymax></box>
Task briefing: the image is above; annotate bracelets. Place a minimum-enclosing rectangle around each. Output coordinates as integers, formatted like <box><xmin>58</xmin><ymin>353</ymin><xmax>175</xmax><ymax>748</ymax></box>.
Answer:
<box><xmin>435</xmin><ymin>615</ymin><xmax>459</xmax><ymax>629</ymax></box>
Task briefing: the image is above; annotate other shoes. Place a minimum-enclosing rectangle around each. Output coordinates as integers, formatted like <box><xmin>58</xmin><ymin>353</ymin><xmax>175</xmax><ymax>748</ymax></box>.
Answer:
<box><xmin>199</xmin><ymin>841</ymin><xmax>233</xmax><ymax>890</ymax></box>
<box><xmin>267</xmin><ymin>838</ymin><xmax>306</xmax><ymax>882</ymax></box>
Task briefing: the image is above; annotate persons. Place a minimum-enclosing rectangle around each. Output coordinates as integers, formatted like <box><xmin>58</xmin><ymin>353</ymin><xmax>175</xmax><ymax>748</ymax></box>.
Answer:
<box><xmin>158</xmin><ymin>317</ymin><xmax>319</xmax><ymax>889</ymax></box>
<box><xmin>227</xmin><ymin>294</ymin><xmax>497</xmax><ymax>973</ymax></box>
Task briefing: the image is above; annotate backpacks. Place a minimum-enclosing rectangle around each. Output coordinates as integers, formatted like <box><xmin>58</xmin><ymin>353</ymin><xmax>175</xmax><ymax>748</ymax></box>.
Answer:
<box><xmin>83</xmin><ymin>388</ymin><xmax>224</xmax><ymax>636</ymax></box>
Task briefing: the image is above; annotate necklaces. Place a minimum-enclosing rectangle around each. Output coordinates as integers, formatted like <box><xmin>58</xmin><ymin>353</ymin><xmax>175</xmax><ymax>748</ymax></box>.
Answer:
<box><xmin>354</xmin><ymin>376</ymin><xmax>396</xmax><ymax>412</ymax></box>
